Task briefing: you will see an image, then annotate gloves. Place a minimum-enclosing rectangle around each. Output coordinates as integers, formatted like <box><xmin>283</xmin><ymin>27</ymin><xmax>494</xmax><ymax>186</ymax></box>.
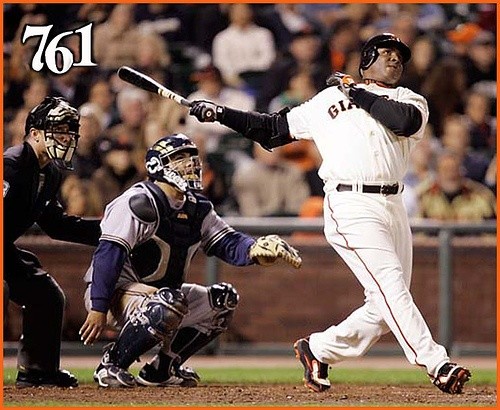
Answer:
<box><xmin>189</xmin><ymin>100</ymin><xmax>225</xmax><ymax>122</ymax></box>
<box><xmin>326</xmin><ymin>72</ymin><xmax>359</xmax><ymax>99</ymax></box>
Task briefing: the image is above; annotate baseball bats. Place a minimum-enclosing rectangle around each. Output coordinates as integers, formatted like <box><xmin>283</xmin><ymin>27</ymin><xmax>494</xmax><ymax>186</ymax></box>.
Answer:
<box><xmin>117</xmin><ymin>65</ymin><xmax>214</xmax><ymax>119</ymax></box>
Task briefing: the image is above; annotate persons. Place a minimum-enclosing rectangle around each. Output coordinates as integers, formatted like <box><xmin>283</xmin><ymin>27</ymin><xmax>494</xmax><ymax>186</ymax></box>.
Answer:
<box><xmin>3</xmin><ymin>95</ymin><xmax>103</xmax><ymax>388</ymax></box>
<box><xmin>77</xmin><ymin>133</ymin><xmax>304</xmax><ymax>389</ymax></box>
<box><xmin>1</xmin><ymin>0</ymin><xmax>499</xmax><ymax>220</ymax></box>
<box><xmin>190</xmin><ymin>32</ymin><xmax>471</xmax><ymax>395</ymax></box>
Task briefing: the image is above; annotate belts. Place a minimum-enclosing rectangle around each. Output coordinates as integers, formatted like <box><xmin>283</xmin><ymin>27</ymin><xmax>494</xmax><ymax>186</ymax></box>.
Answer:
<box><xmin>336</xmin><ymin>182</ymin><xmax>407</xmax><ymax>193</ymax></box>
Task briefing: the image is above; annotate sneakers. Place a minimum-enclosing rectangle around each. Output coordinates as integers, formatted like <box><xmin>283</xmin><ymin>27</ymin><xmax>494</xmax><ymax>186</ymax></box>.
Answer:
<box><xmin>434</xmin><ymin>363</ymin><xmax>472</xmax><ymax>393</ymax></box>
<box><xmin>136</xmin><ymin>362</ymin><xmax>200</xmax><ymax>385</ymax></box>
<box><xmin>89</xmin><ymin>354</ymin><xmax>137</xmax><ymax>388</ymax></box>
<box><xmin>15</xmin><ymin>370</ymin><xmax>81</xmax><ymax>388</ymax></box>
<box><xmin>293</xmin><ymin>335</ymin><xmax>333</xmax><ymax>392</ymax></box>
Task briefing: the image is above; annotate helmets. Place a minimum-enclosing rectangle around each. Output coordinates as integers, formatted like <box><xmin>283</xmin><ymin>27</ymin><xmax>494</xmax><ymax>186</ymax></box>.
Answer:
<box><xmin>24</xmin><ymin>96</ymin><xmax>80</xmax><ymax>171</ymax></box>
<box><xmin>146</xmin><ymin>133</ymin><xmax>203</xmax><ymax>194</ymax></box>
<box><xmin>358</xmin><ymin>33</ymin><xmax>410</xmax><ymax>76</ymax></box>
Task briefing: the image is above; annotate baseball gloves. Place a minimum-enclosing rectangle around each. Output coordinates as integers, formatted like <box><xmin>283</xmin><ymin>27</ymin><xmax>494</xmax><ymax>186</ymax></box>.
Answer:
<box><xmin>249</xmin><ymin>234</ymin><xmax>302</xmax><ymax>269</ymax></box>
